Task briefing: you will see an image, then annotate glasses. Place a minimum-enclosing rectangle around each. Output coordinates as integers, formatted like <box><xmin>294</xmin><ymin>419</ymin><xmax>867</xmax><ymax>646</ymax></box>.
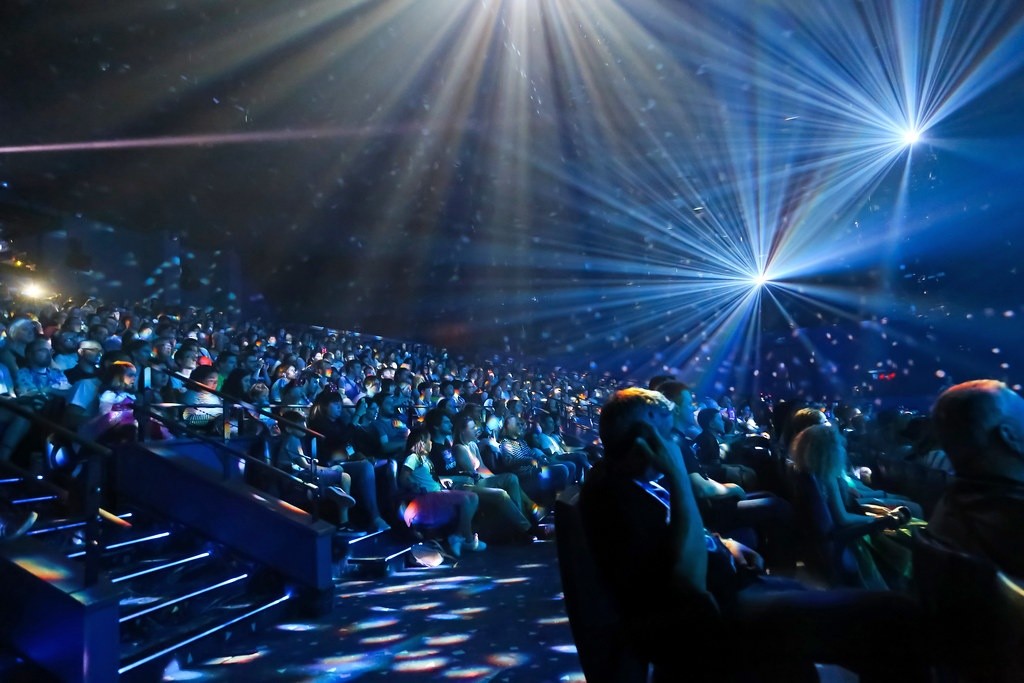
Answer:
<box><xmin>31</xmin><ymin>348</ymin><xmax>56</xmax><ymax>356</ymax></box>
<box><xmin>63</xmin><ymin>338</ymin><xmax>78</xmax><ymax>345</ymax></box>
<box><xmin>82</xmin><ymin>347</ymin><xmax>105</xmax><ymax>355</ymax></box>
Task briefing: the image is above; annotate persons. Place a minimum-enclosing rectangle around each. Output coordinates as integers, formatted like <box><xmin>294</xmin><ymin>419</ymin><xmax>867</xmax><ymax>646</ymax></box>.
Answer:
<box><xmin>928</xmin><ymin>379</ymin><xmax>1024</xmax><ymax>580</ymax></box>
<box><xmin>0</xmin><ymin>241</ymin><xmax>953</xmax><ymax>611</ymax></box>
<box><xmin>580</xmin><ymin>387</ymin><xmax>923</xmax><ymax>683</ymax></box>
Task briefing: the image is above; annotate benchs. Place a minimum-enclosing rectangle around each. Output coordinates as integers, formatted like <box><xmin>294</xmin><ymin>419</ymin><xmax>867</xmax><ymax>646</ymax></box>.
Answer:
<box><xmin>140</xmin><ymin>437</ymin><xmax>445</xmax><ymax>581</ymax></box>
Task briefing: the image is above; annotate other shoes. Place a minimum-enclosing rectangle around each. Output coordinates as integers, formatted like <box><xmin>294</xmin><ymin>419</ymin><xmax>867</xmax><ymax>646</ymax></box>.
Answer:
<box><xmin>532</xmin><ymin>525</ymin><xmax>552</xmax><ymax>539</ymax></box>
<box><xmin>369</xmin><ymin>516</ymin><xmax>392</xmax><ymax>532</ymax></box>
<box><xmin>463</xmin><ymin>533</ymin><xmax>487</xmax><ymax>551</ymax></box>
<box><xmin>447</xmin><ymin>532</ymin><xmax>463</xmax><ymax>557</ymax></box>
<box><xmin>518</xmin><ymin>532</ymin><xmax>537</xmax><ymax>543</ymax></box>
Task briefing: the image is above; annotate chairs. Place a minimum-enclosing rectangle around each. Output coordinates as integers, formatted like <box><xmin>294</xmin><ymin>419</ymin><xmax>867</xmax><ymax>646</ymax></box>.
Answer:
<box><xmin>555</xmin><ymin>488</ymin><xmax>820</xmax><ymax>683</ymax></box>
<box><xmin>793</xmin><ymin>473</ymin><xmax>912</xmax><ymax>589</ymax></box>
<box><xmin>911</xmin><ymin>526</ymin><xmax>1024</xmax><ymax>683</ymax></box>
